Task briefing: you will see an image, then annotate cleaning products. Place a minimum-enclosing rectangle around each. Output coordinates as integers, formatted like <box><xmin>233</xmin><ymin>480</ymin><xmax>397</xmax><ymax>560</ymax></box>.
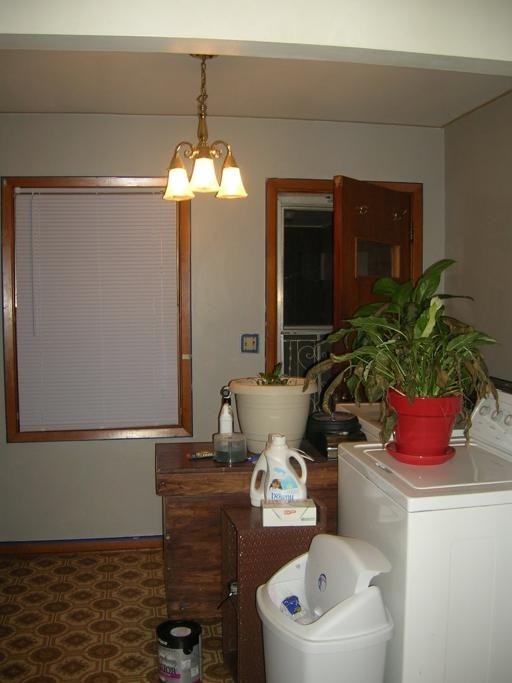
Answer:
<box><xmin>219</xmin><ymin>386</ymin><xmax>235</xmax><ymax>435</ymax></box>
<box><xmin>251</xmin><ymin>435</ymin><xmax>309</xmax><ymax>507</ymax></box>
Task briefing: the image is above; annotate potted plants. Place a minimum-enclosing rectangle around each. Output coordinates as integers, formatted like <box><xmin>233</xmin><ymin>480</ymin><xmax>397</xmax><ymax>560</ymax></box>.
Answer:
<box><xmin>298</xmin><ymin>255</ymin><xmax>501</xmax><ymax>467</ymax></box>
<box><xmin>228</xmin><ymin>361</ymin><xmax>318</xmax><ymax>455</ymax></box>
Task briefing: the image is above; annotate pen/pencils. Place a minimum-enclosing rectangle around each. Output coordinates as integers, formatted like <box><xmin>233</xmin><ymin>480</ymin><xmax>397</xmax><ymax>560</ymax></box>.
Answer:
<box><xmin>191</xmin><ymin>451</ymin><xmax>213</xmax><ymax>460</ymax></box>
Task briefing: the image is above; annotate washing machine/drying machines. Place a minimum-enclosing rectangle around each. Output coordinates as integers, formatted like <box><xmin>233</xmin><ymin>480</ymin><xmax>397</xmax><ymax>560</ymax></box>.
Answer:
<box><xmin>339</xmin><ymin>388</ymin><xmax>511</xmax><ymax>682</ymax></box>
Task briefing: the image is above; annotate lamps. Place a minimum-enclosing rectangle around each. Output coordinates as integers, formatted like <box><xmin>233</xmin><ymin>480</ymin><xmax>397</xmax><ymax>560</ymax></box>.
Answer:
<box><xmin>161</xmin><ymin>54</ymin><xmax>250</xmax><ymax>201</ymax></box>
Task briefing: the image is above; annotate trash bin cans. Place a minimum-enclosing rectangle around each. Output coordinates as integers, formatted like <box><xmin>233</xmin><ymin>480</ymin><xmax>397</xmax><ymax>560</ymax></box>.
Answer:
<box><xmin>256</xmin><ymin>533</ymin><xmax>394</xmax><ymax>683</ymax></box>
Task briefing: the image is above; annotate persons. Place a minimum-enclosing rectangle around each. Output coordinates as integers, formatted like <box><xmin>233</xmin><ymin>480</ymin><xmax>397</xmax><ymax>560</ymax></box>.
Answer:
<box><xmin>268</xmin><ymin>477</ymin><xmax>284</xmax><ymax>490</ymax></box>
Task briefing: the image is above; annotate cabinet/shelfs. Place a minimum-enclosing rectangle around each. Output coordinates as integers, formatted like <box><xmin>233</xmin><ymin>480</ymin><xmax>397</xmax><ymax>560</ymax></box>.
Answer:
<box><xmin>219</xmin><ymin>504</ymin><xmax>328</xmax><ymax>683</ymax></box>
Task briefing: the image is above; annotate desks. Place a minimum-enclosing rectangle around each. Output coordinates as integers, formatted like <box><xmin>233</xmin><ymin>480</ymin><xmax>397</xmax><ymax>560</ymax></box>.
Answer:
<box><xmin>154</xmin><ymin>438</ymin><xmax>340</xmax><ymax>625</ymax></box>
